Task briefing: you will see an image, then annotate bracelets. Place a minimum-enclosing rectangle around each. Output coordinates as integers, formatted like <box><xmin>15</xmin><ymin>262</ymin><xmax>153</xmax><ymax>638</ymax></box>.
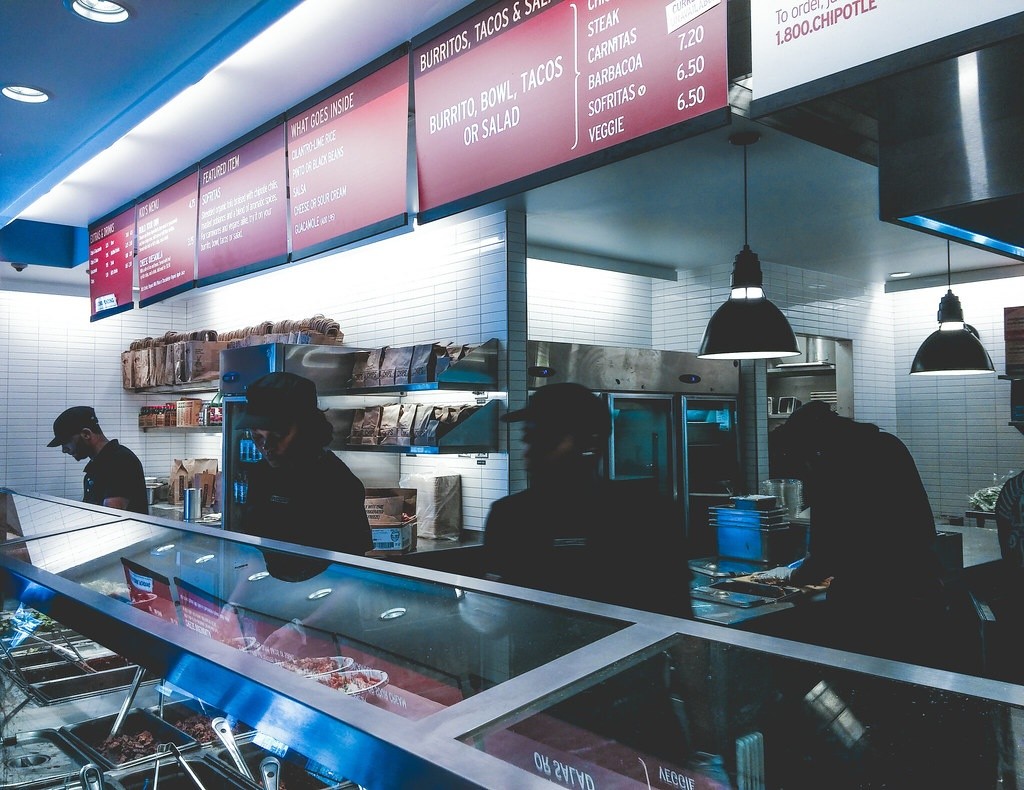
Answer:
<box><xmin>291</xmin><ymin>618</ymin><xmax>306</xmax><ymax>646</ymax></box>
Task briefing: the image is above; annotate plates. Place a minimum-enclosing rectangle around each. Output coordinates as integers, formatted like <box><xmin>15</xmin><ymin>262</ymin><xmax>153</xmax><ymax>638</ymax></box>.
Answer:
<box><xmin>217</xmin><ymin>636</ymin><xmax>389</xmax><ymax>702</ymax></box>
<box><xmin>126</xmin><ymin>592</ymin><xmax>157</xmax><ymax>610</ymax></box>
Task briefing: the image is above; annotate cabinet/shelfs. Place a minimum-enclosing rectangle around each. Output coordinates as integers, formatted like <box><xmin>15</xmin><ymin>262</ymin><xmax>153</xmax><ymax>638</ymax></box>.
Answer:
<box><xmin>284</xmin><ymin>349</ymin><xmax>498</xmax><ymax>561</ymax></box>
<box><xmin>998</xmin><ymin>306</ymin><xmax>1024</xmax><ymax>437</ymax></box>
<box><xmin>119</xmin><ymin>325</ymin><xmax>222</xmax><ymax>519</ymax></box>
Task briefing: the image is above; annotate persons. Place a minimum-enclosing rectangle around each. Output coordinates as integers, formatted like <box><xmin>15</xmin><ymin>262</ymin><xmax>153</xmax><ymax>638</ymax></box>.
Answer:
<box><xmin>460</xmin><ymin>385</ymin><xmax>730</xmax><ymax>790</ymax></box>
<box><xmin>750</xmin><ymin>400</ymin><xmax>943</xmax><ymax>657</ymax></box>
<box><xmin>211</xmin><ymin>372</ymin><xmax>375</xmax><ymax>659</ymax></box>
<box><xmin>47</xmin><ymin>406</ymin><xmax>148</xmax><ymax>516</ymax></box>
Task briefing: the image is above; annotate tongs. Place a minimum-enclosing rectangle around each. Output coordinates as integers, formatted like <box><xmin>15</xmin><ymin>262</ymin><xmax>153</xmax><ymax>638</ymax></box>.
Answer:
<box><xmin>152</xmin><ymin>742</ymin><xmax>207</xmax><ymax>790</ymax></box>
<box><xmin>108</xmin><ymin>667</ymin><xmax>166</xmax><ymax>742</ymax></box>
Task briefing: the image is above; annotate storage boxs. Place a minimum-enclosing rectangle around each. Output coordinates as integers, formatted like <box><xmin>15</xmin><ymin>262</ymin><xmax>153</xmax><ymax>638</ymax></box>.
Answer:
<box><xmin>364</xmin><ymin>495</ymin><xmax>419</xmax><ymax>551</ymax></box>
<box><xmin>709</xmin><ymin>494</ymin><xmax>811</xmax><ymax>564</ymax></box>
<box><xmin>686</xmin><ymin>421</ymin><xmax>720</xmax><ymax>443</ymax></box>
<box><xmin>935</xmin><ymin>532</ymin><xmax>964</xmax><ymax>578</ymax></box>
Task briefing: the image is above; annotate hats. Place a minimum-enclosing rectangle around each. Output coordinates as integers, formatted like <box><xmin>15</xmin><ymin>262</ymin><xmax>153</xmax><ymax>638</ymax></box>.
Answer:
<box><xmin>234</xmin><ymin>371</ymin><xmax>315</xmax><ymax>431</ymax></box>
<box><xmin>47</xmin><ymin>406</ymin><xmax>94</xmax><ymax>447</ymax></box>
<box><xmin>500</xmin><ymin>382</ymin><xmax>612</xmax><ymax>438</ymax></box>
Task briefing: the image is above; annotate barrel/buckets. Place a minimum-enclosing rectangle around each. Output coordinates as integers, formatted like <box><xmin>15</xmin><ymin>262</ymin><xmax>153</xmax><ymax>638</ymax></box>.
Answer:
<box><xmin>806</xmin><ymin>337</ymin><xmax>836</xmax><ymax>365</ymax></box>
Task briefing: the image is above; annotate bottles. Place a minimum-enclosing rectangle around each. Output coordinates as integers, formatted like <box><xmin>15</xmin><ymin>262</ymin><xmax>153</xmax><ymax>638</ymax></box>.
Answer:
<box><xmin>139</xmin><ymin>403</ymin><xmax>176</xmax><ymax>427</ymax></box>
<box><xmin>239</xmin><ymin>428</ymin><xmax>262</xmax><ymax>463</ymax></box>
<box><xmin>233</xmin><ymin>472</ymin><xmax>248</xmax><ymax>504</ymax></box>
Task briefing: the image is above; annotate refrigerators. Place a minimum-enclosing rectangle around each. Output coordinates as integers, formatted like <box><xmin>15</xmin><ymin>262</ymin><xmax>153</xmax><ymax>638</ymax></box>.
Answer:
<box><xmin>218</xmin><ymin>340</ymin><xmax>400</xmax><ymax>531</ymax></box>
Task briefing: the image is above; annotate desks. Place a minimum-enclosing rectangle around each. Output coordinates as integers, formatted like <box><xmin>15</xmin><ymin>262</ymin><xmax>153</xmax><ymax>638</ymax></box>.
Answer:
<box><xmin>967</xmin><ymin>510</ymin><xmax>998</xmax><ymax>529</ymax></box>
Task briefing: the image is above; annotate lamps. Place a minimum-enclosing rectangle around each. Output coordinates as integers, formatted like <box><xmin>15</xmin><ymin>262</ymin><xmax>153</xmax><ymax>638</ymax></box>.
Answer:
<box><xmin>696</xmin><ymin>128</ymin><xmax>801</xmax><ymax>361</ymax></box>
<box><xmin>906</xmin><ymin>235</ymin><xmax>995</xmax><ymax>375</ymax></box>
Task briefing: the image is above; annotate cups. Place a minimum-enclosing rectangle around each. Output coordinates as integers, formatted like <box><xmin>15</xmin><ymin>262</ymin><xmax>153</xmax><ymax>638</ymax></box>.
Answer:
<box><xmin>184</xmin><ymin>488</ymin><xmax>202</xmax><ymax>520</ymax></box>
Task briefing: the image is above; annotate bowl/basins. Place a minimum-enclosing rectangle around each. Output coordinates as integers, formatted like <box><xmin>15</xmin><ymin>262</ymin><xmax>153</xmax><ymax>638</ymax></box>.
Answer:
<box><xmin>761</xmin><ymin>479</ymin><xmax>805</xmax><ymax>522</ymax></box>
<box><xmin>146</xmin><ymin>483</ymin><xmax>164</xmax><ymax>505</ymax></box>
